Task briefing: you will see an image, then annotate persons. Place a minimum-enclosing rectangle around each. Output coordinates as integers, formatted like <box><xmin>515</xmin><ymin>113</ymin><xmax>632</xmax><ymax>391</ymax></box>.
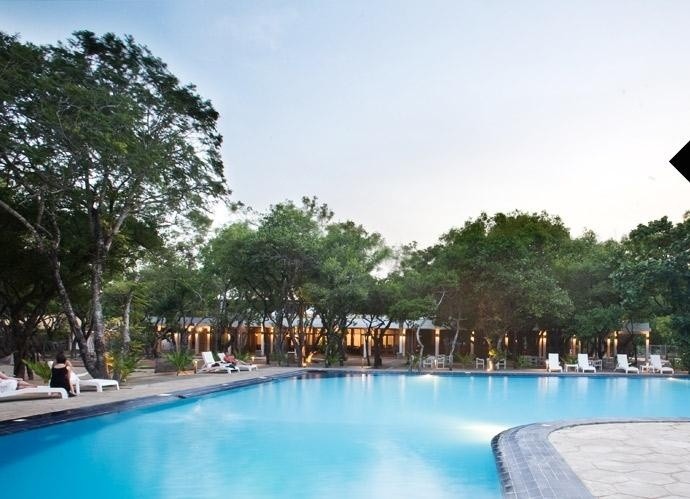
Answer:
<box><xmin>1</xmin><ymin>372</ymin><xmax>38</xmax><ymax>392</ymax></box>
<box><xmin>224</xmin><ymin>352</ymin><xmax>252</xmax><ymax>365</ymax></box>
<box><xmin>50</xmin><ymin>354</ymin><xmax>76</xmax><ymax>397</ymax></box>
<box><xmin>53</xmin><ymin>355</ymin><xmax>77</xmax><ymax>396</ymax></box>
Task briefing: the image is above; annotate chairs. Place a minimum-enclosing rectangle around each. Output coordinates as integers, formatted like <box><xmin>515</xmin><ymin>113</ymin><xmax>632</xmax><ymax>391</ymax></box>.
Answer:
<box><xmin>545</xmin><ymin>352</ymin><xmax>674</xmax><ymax>375</ymax></box>
<box><xmin>196</xmin><ymin>351</ymin><xmax>258</xmax><ymax>375</ymax></box>
<box><xmin>0</xmin><ymin>360</ymin><xmax>119</xmax><ymax>399</ymax></box>
